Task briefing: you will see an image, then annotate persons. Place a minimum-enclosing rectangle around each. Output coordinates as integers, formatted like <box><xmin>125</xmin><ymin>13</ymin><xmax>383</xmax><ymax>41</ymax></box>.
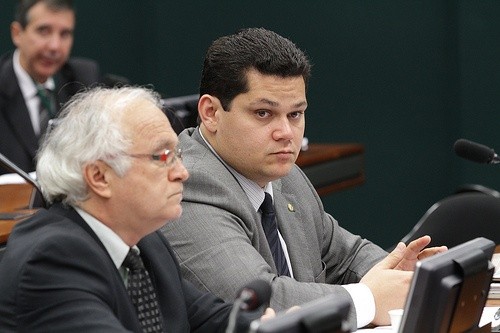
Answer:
<box><xmin>162</xmin><ymin>30</ymin><xmax>448</xmax><ymax>327</ymax></box>
<box><xmin>0</xmin><ymin>0</ymin><xmax>103</xmax><ymax>173</ymax></box>
<box><xmin>0</xmin><ymin>87</ymin><xmax>300</xmax><ymax>333</ymax></box>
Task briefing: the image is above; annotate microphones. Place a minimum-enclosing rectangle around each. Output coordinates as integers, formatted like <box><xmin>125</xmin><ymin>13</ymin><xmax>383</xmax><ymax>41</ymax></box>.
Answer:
<box><xmin>454</xmin><ymin>139</ymin><xmax>500</xmax><ymax>165</ymax></box>
<box><xmin>224</xmin><ymin>279</ymin><xmax>273</xmax><ymax>333</ymax></box>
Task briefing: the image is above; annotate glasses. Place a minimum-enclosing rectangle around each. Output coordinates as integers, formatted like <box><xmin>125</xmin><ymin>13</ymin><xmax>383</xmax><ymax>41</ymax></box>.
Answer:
<box><xmin>113</xmin><ymin>143</ymin><xmax>186</xmax><ymax>166</ymax></box>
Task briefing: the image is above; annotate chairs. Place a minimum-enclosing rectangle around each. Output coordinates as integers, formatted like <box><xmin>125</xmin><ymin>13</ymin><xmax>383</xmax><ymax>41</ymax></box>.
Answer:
<box><xmin>398</xmin><ymin>191</ymin><xmax>500</xmax><ymax>253</ymax></box>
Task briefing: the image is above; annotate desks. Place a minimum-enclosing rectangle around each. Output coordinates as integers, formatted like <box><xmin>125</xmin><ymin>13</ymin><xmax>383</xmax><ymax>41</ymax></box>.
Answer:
<box><xmin>0</xmin><ymin>142</ymin><xmax>368</xmax><ymax>246</ymax></box>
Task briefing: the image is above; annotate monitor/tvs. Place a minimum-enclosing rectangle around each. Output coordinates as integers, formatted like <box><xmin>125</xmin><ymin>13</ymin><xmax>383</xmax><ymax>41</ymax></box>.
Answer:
<box><xmin>160</xmin><ymin>94</ymin><xmax>202</xmax><ymax>134</ymax></box>
<box><xmin>398</xmin><ymin>237</ymin><xmax>496</xmax><ymax>333</ymax></box>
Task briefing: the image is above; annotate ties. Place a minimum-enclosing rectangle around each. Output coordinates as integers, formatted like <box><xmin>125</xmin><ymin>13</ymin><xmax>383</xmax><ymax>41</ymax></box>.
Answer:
<box><xmin>124</xmin><ymin>247</ymin><xmax>162</xmax><ymax>333</ymax></box>
<box><xmin>259</xmin><ymin>191</ymin><xmax>290</xmax><ymax>278</ymax></box>
<box><xmin>37</xmin><ymin>88</ymin><xmax>55</xmax><ymax>138</ymax></box>
